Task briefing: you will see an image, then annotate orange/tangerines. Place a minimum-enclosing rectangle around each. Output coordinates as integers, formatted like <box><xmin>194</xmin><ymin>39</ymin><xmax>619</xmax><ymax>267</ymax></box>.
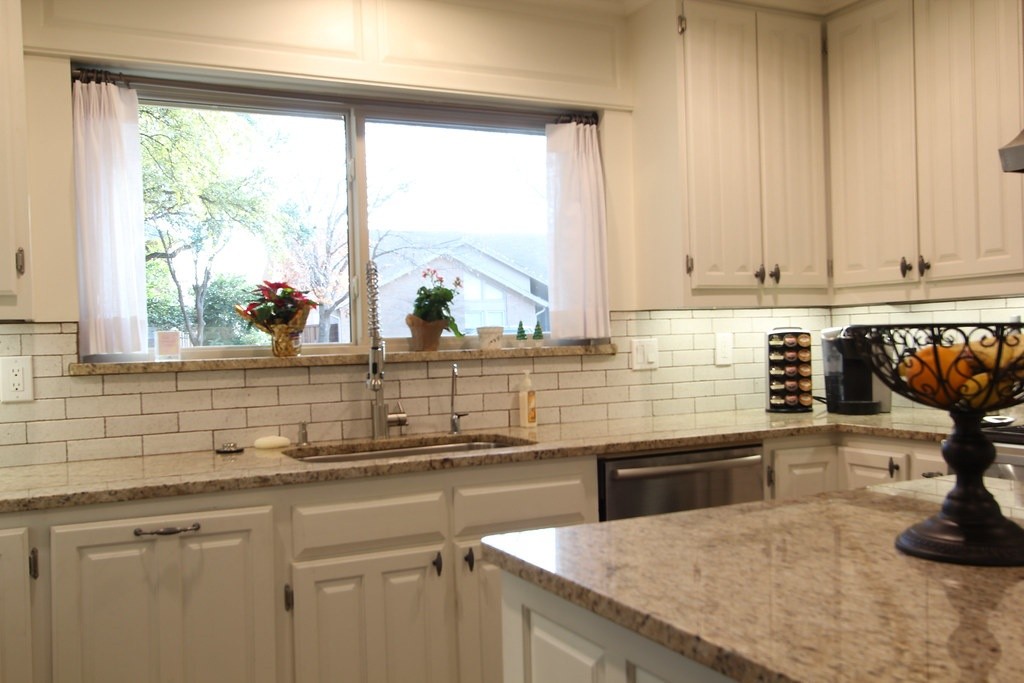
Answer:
<box><xmin>907</xmin><ymin>346</ymin><xmax>971</xmax><ymax>405</ymax></box>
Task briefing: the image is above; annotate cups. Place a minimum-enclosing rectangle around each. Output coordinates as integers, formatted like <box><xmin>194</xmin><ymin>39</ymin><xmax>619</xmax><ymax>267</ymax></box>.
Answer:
<box><xmin>154</xmin><ymin>331</ymin><xmax>180</xmax><ymax>361</ymax></box>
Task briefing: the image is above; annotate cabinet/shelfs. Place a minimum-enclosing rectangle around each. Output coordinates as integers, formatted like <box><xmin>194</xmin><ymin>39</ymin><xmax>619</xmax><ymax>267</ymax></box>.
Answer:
<box><xmin>480</xmin><ymin>473</ymin><xmax>1024</xmax><ymax>683</ymax></box>
<box><xmin>0</xmin><ymin>0</ymin><xmax>33</xmax><ymax>320</ymax></box>
<box><xmin>1</xmin><ymin>458</ymin><xmax>600</xmax><ymax>683</ymax></box>
<box><xmin>761</xmin><ymin>432</ymin><xmax>1024</xmax><ymax>499</ymax></box>
<box><xmin>625</xmin><ymin>2</ymin><xmax>1023</xmax><ymax>311</ymax></box>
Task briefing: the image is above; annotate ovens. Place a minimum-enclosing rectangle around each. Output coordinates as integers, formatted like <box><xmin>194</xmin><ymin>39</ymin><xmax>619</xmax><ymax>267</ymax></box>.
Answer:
<box><xmin>599</xmin><ymin>444</ymin><xmax>764</xmax><ymax>521</ymax></box>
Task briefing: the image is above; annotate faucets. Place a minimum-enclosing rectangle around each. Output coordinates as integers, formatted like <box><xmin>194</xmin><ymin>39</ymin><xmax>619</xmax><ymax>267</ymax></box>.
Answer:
<box><xmin>364</xmin><ymin>257</ymin><xmax>408</xmax><ymax>441</ymax></box>
<box><xmin>450</xmin><ymin>362</ymin><xmax>469</xmax><ymax>437</ymax></box>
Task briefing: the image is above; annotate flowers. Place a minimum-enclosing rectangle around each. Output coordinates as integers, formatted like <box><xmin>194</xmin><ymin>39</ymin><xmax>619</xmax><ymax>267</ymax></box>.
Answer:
<box><xmin>242</xmin><ymin>281</ymin><xmax>320</xmax><ymax>334</ymax></box>
<box><xmin>413</xmin><ymin>268</ymin><xmax>467</xmax><ymax>337</ymax></box>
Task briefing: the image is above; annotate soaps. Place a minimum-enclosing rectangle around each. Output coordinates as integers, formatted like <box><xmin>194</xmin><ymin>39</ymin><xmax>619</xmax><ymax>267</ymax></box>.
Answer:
<box><xmin>254</xmin><ymin>434</ymin><xmax>290</xmax><ymax>449</ymax></box>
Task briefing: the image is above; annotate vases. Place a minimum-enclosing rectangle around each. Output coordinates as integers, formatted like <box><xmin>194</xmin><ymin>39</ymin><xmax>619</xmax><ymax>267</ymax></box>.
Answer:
<box><xmin>405</xmin><ymin>314</ymin><xmax>449</xmax><ymax>352</ymax></box>
<box><xmin>236</xmin><ymin>306</ymin><xmax>309</xmax><ymax>356</ymax></box>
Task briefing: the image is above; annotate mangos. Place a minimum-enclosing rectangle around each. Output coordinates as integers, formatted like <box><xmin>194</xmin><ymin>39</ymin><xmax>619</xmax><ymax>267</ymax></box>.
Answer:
<box><xmin>959</xmin><ymin>372</ymin><xmax>1014</xmax><ymax>410</ymax></box>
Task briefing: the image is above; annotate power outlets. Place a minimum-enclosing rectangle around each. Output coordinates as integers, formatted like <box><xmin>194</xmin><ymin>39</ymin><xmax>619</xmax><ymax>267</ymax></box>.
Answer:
<box><xmin>0</xmin><ymin>356</ymin><xmax>34</xmax><ymax>404</ymax></box>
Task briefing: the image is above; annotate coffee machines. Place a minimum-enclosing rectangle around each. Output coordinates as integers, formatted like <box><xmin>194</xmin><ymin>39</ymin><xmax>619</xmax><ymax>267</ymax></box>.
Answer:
<box><xmin>822</xmin><ymin>325</ymin><xmax>892</xmax><ymax>416</ymax></box>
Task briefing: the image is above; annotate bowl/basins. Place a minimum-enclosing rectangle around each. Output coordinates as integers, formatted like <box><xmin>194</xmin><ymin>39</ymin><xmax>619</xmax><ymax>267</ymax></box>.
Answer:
<box><xmin>477</xmin><ymin>327</ymin><xmax>503</xmax><ymax>350</ymax></box>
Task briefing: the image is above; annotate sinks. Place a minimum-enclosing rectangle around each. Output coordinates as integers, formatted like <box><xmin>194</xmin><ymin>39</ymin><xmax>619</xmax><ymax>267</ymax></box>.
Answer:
<box><xmin>281</xmin><ymin>433</ymin><xmax>538</xmax><ymax>464</ymax></box>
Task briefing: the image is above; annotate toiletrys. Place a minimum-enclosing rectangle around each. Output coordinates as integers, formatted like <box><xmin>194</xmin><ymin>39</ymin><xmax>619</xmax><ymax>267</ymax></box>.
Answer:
<box><xmin>517</xmin><ymin>370</ymin><xmax>537</xmax><ymax>429</ymax></box>
